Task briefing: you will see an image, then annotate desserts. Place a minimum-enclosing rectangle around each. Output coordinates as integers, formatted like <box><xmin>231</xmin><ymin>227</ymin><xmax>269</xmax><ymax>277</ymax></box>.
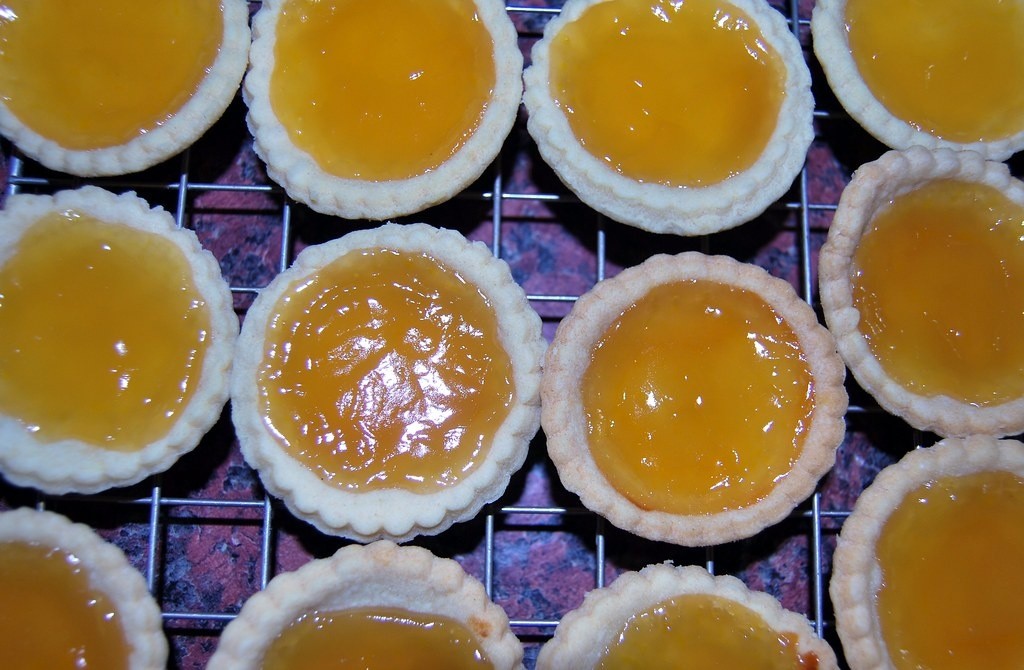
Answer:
<box><xmin>0</xmin><ymin>1</ymin><xmax>1024</xmax><ymax>670</ymax></box>
